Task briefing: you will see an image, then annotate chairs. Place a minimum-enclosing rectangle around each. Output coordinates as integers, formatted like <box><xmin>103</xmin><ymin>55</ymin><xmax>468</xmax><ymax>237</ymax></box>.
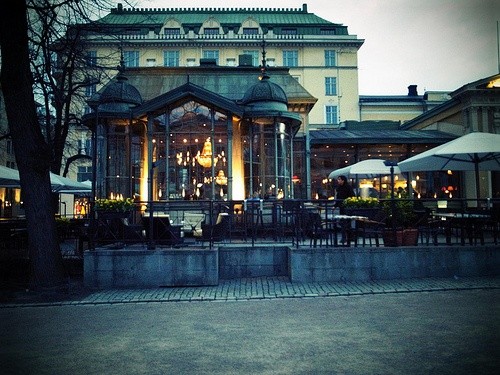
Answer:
<box><xmin>0</xmin><ymin>222</ymin><xmax>18</xmax><ymax>248</ymax></box>
<box><xmin>409</xmin><ymin>206</ymin><xmax>500</xmax><ymax>247</ymax></box>
<box><xmin>120</xmin><ymin>210</ymin><xmax>205</xmax><ymax>247</ymax></box>
<box><xmin>199</xmin><ymin>199</ymin><xmax>386</xmax><ymax>247</ymax></box>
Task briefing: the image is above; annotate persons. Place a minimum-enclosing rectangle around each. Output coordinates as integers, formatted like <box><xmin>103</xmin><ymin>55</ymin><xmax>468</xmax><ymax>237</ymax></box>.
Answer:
<box><xmin>331</xmin><ymin>175</ymin><xmax>355</xmax><ymax>243</ymax></box>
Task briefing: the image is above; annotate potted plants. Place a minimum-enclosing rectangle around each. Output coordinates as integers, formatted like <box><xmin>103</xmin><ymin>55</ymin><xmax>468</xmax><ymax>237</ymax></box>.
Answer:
<box><xmin>383</xmin><ymin>189</ymin><xmax>421</xmax><ymax>247</ymax></box>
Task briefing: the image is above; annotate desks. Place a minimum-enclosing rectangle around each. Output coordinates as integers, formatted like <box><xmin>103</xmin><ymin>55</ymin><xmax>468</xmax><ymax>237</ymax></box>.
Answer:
<box><xmin>431</xmin><ymin>212</ymin><xmax>492</xmax><ymax>245</ymax></box>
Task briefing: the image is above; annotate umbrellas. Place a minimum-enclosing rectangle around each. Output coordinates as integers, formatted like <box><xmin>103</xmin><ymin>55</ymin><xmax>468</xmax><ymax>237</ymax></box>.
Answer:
<box><xmin>0</xmin><ymin>165</ymin><xmax>21</xmax><ymax>188</ymax></box>
<box><xmin>327</xmin><ymin>157</ymin><xmax>403</xmax><ymax>199</ymax></box>
<box><xmin>48</xmin><ymin>170</ymin><xmax>92</xmax><ymax>194</ymax></box>
<box><xmin>397</xmin><ymin>130</ymin><xmax>500</xmax><ymax>207</ymax></box>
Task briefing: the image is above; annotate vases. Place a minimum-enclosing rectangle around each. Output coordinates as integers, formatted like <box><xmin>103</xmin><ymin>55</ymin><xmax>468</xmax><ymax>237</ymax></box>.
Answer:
<box><xmin>57</xmin><ymin>223</ymin><xmax>67</xmax><ymax>244</ymax></box>
<box><xmin>348</xmin><ymin>206</ymin><xmax>378</xmax><ymax>226</ymax></box>
<box><xmin>97</xmin><ymin>213</ymin><xmax>128</xmax><ymax>240</ymax></box>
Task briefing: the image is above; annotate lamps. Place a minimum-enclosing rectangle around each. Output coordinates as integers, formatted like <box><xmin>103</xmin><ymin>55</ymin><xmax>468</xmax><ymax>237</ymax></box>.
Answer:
<box><xmin>195</xmin><ymin>135</ymin><xmax>218</xmax><ymax>167</ymax></box>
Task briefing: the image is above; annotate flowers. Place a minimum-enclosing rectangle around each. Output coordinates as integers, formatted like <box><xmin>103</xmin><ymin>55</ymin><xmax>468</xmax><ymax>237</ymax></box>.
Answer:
<box><xmin>93</xmin><ymin>198</ymin><xmax>139</xmax><ymax>212</ymax></box>
<box><xmin>342</xmin><ymin>196</ymin><xmax>382</xmax><ymax>208</ymax></box>
<box><xmin>56</xmin><ymin>215</ymin><xmax>70</xmax><ymax>226</ymax></box>
